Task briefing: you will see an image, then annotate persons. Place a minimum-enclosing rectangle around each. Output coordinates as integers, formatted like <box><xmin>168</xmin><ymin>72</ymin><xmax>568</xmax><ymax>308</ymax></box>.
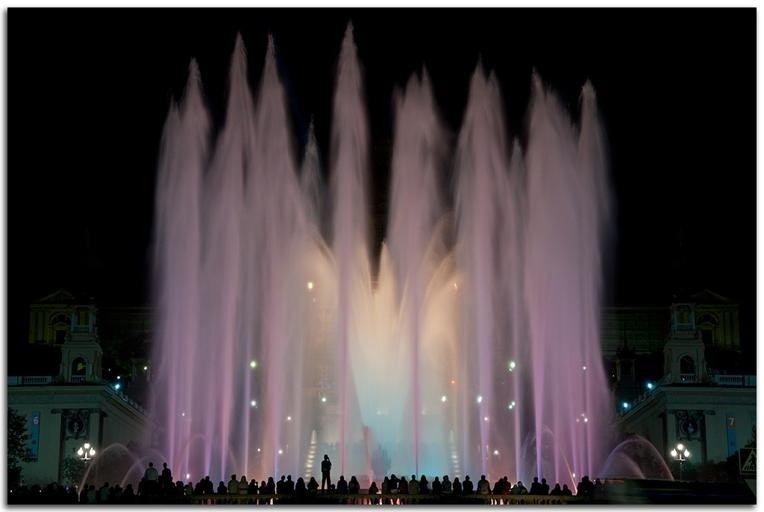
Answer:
<box><xmin>161</xmin><ymin>462</ymin><xmax>171</xmax><ymax>480</ymax></box>
<box><xmin>11</xmin><ymin>472</ymin><xmax>609</xmax><ymax>504</ymax></box>
<box><xmin>321</xmin><ymin>454</ymin><xmax>331</xmax><ymax>489</ymax></box>
<box><xmin>142</xmin><ymin>462</ymin><xmax>158</xmax><ymax>481</ymax></box>
